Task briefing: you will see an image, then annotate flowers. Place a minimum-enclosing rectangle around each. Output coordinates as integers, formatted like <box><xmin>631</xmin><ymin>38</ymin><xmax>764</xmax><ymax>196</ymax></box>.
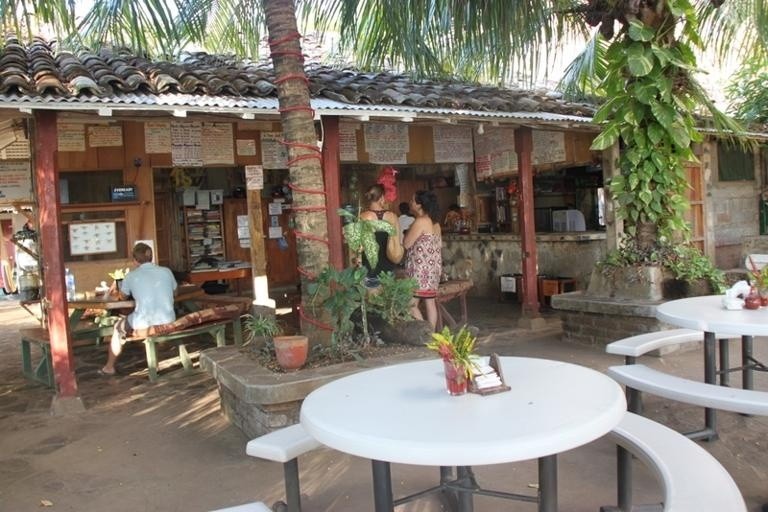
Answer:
<box><xmin>423</xmin><ymin>323</ymin><xmax>484</xmax><ymax>382</ymax></box>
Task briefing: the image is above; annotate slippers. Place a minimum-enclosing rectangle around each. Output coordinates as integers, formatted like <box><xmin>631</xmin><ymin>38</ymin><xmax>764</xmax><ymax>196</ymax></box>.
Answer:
<box><xmin>95</xmin><ymin>368</ymin><xmax>116</xmax><ymax>375</ymax></box>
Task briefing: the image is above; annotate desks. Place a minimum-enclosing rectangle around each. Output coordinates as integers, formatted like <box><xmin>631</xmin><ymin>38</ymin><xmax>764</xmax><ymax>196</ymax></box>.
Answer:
<box><xmin>300</xmin><ymin>355</ymin><xmax>627</xmax><ymax>511</ymax></box>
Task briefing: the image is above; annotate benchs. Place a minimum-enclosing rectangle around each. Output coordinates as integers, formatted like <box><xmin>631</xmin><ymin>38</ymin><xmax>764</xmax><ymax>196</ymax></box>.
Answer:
<box><xmin>605</xmin><ymin>254</ymin><xmax>768</xmax><ymax>510</ymax></box>
<box><xmin>211</xmin><ymin>422</ymin><xmax>324</xmax><ymax>512</ymax></box>
<box><xmin>282</xmin><ymin>269</ymin><xmax>474</xmax><ymax>346</ymax></box>
<box><xmin>20</xmin><ymin>266</ymin><xmax>253</xmax><ymax>387</ymax></box>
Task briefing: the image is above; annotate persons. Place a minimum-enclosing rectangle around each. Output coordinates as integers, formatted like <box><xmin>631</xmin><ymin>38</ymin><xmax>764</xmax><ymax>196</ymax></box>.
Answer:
<box><xmin>397</xmin><ymin>201</ymin><xmax>415</xmax><ymax>244</ymax></box>
<box><xmin>356</xmin><ymin>183</ymin><xmax>405</xmax><ymax>285</ymax></box>
<box><xmin>444</xmin><ymin>204</ymin><xmax>460</xmax><ymax>226</ymax></box>
<box><xmin>97</xmin><ymin>243</ymin><xmax>177</xmax><ymax>377</ymax></box>
<box><xmin>404</xmin><ymin>190</ymin><xmax>443</xmax><ymax>332</ymax></box>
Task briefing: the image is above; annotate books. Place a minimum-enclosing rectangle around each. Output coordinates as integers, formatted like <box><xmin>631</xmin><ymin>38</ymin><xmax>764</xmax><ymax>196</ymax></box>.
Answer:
<box><xmin>181</xmin><ymin>209</ymin><xmax>252</xmax><ymax>271</ymax></box>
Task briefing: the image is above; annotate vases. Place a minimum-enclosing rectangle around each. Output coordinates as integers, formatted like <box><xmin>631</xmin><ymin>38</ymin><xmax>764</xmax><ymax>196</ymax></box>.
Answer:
<box><xmin>444</xmin><ymin>358</ymin><xmax>469</xmax><ymax>398</ymax></box>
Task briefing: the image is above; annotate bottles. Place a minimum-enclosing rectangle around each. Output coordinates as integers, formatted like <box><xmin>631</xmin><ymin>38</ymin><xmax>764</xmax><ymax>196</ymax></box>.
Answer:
<box><xmin>744</xmin><ymin>283</ymin><xmax>760</xmax><ymax>310</ymax></box>
<box><xmin>65</xmin><ymin>268</ymin><xmax>76</xmax><ymax>302</ymax></box>
<box><xmin>460</xmin><ymin>227</ymin><xmax>471</xmax><ymax>235</ymax></box>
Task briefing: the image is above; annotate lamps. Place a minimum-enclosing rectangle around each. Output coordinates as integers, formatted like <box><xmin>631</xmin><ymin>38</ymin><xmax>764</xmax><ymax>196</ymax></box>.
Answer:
<box><xmin>475</xmin><ymin>124</ymin><xmax>485</xmax><ymax>136</ymax></box>
